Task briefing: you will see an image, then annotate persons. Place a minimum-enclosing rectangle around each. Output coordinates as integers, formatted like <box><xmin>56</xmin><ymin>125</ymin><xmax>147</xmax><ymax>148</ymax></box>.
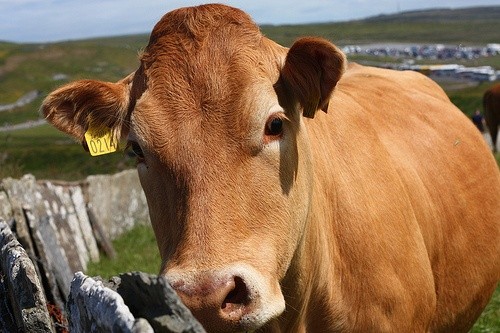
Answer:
<box><xmin>472</xmin><ymin>110</ymin><xmax>484</xmax><ymax>131</ymax></box>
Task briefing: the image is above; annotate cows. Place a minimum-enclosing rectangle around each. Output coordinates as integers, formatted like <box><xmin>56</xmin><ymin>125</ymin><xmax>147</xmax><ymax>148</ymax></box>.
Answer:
<box><xmin>37</xmin><ymin>2</ymin><xmax>500</xmax><ymax>333</ymax></box>
<box><xmin>482</xmin><ymin>85</ymin><xmax>500</xmax><ymax>152</ymax></box>
